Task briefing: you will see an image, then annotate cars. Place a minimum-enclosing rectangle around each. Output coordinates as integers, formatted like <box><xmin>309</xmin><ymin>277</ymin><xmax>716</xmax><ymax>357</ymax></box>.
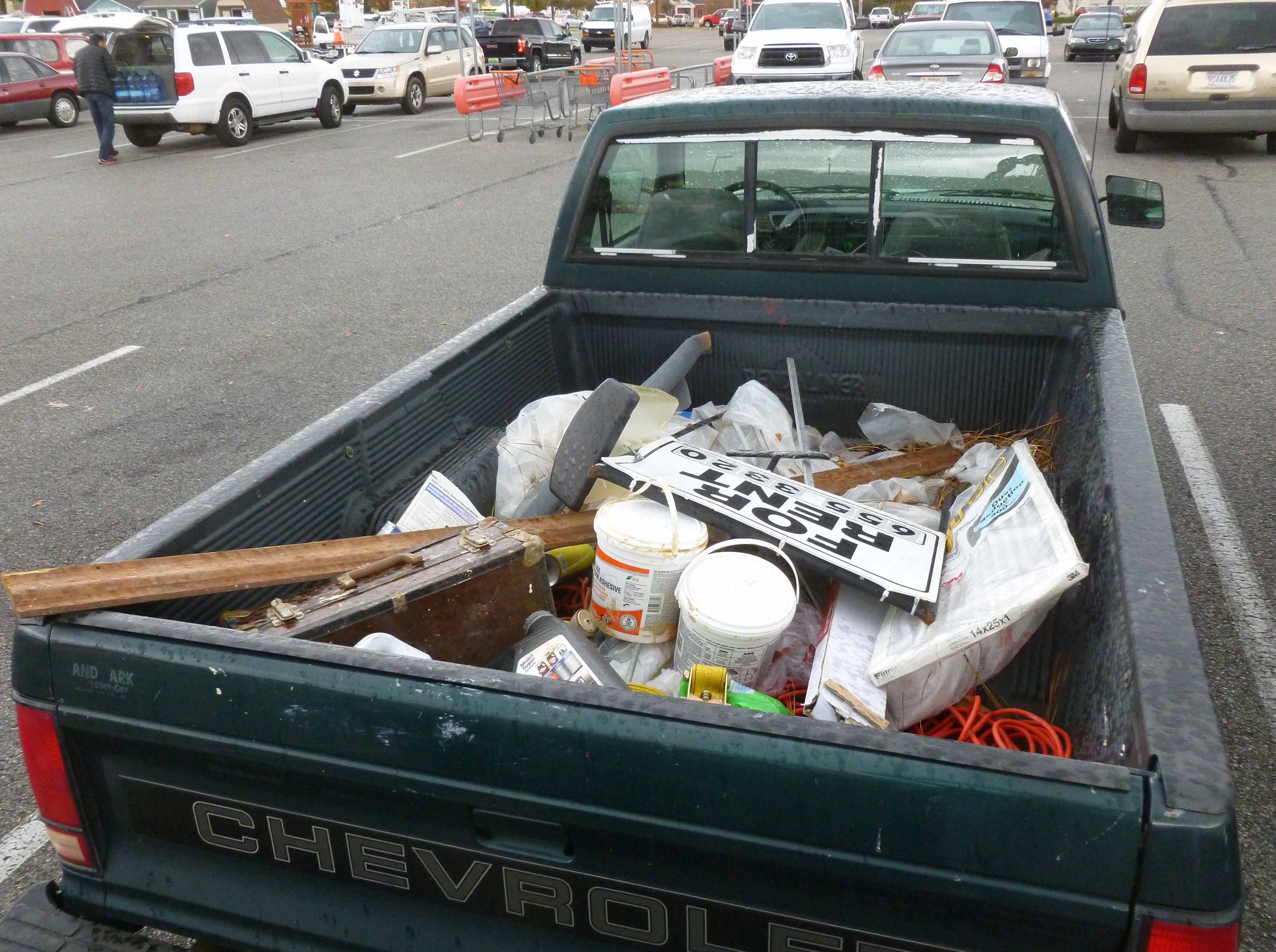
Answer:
<box><xmin>332</xmin><ymin>22</ymin><xmax>487</xmax><ymax>116</ymax></box>
<box><xmin>1105</xmin><ymin>0</ymin><xmax>1276</xmax><ymax>155</ymax></box>
<box><xmin>0</xmin><ymin>2</ymin><xmax>654</xmax><ymax>124</ymax></box>
<box><xmin>1096</xmin><ymin>4</ymin><xmax>1146</xmax><ymax>23</ymax></box>
<box><xmin>699</xmin><ymin>8</ymin><xmax>733</xmax><ymax>28</ymax></box>
<box><xmin>867</xmin><ymin>20</ymin><xmax>1019</xmax><ymax>84</ymax></box>
<box><xmin>1043</xmin><ymin>8</ymin><xmax>1055</xmax><ymax>27</ymax></box>
<box><xmin>1073</xmin><ymin>5</ymin><xmax>1096</xmax><ymax>17</ymax></box>
<box><xmin>1064</xmin><ymin>12</ymin><xmax>1132</xmax><ymax>62</ymax></box>
<box><xmin>0</xmin><ymin>51</ymin><xmax>81</xmax><ymax>128</ymax></box>
<box><xmin>904</xmin><ymin>1</ymin><xmax>948</xmax><ymax>22</ymax></box>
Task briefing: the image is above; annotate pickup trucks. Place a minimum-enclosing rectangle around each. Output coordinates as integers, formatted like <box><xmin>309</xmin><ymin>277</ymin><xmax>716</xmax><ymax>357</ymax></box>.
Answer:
<box><xmin>719</xmin><ymin>2</ymin><xmax>763</xmax><ymax>51</ymax></box>
<box><xmin>8</xmin><ymin>79</ymin><xmax>1246</xmax><ymax>952</ymax></box>
<box><xmin>868</xmin><ymin>6</ymin><xmax>910</xmax><ymax>29</ymax></box>
<box><xmin>476</xmin><ymin>16</ymin><xmax>582</xmax><ymax>83</ymax></box>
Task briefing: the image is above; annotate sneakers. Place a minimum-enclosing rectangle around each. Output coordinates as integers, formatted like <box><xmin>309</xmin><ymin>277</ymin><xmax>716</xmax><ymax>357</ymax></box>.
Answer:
<box><xmin>108</xmin><ymin>149</ymin><xmax>119</xmax><ymax>156</ymax></box>
<box><xmin>97</xmin><ymin>157</ymin><xmax>117</xmax><ymax>164</ymax></box>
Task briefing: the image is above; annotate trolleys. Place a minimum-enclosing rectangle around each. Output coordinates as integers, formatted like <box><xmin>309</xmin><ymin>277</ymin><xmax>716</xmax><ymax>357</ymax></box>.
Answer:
<box><xmin>490</xmin><ymin>66</ymin><xmax>568</xmax><ymax>144</ymax></box>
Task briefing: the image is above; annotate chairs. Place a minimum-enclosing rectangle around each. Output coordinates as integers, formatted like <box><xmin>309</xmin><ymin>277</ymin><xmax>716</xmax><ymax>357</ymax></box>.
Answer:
<box><xmin>882</xmin><ymin>210</ymin><xmax>1012</xmax><ymax>260</ymax></box>
<box><xmin>899</xmin><ymin>45</ymin><xmax>920</xmax><ymax>55</ymax></box>
<box><xmin>960</xmin><ymin>13</ymin><xmax>973</xmax><ymax>21</ymax></box>
<box><xmin>637</xmin><ymin>189</ymin><xmax>745</xmax><ymax>252</ymax></box>
<box><xmin>964</xmin><ymin>39</ymin><xmax>980</xmax><ymax>45</ymax></box>
<box><xmin>960</xmin><ymin>45</ymin><xmax>981</xmax><ymax>55</ymax></box>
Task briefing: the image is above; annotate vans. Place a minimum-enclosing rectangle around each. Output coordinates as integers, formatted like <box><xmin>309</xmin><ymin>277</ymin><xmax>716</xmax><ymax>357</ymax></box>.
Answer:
<box><xmin>940</xmin><ymin>0</ymin><xmax>1065</xmax><ymax>88</ymax></box>
<box><xmin>581</xmin><ymin>0</ymin><xmax>653</xmax><ymax>53</ymax></box>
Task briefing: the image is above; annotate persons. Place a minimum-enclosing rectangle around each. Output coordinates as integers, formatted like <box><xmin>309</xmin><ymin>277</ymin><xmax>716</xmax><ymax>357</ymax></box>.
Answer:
<box><xmin>71</xmin><ymin>34</ymin><xmax>119</xmax><ymax>164</ymax></box>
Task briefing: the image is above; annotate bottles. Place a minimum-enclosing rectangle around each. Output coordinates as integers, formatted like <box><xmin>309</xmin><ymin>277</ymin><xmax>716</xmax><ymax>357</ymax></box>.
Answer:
<box><xmin>126</xmin><ymin>69</ymin><xmax>146</xmax><ymax>102</ymax></box>
<box><xmin>679</xmin><ymin>671</ymin><xmax>795</xmax><ymax>715</ymax></box>
<box><xmin>112</xmin><ymin>68</ymin><xmax>131</xmax><ymax>103</ymax></box>
<box><xmin>493</xmin><ymin>611</ymin><xmax>632</xmax><ymax>692</ymax></box>
<box><xmin>142</xmin><ymin>68</ymin><xmax>165</xmax><ymax>103</ymax></box>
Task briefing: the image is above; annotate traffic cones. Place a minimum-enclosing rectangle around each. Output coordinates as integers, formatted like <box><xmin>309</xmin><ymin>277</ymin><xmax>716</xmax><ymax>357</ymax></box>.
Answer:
<box><xmin>332</xmin><ymin>18</ymin><xmax>345</xmax><ymax>45</ymax></box>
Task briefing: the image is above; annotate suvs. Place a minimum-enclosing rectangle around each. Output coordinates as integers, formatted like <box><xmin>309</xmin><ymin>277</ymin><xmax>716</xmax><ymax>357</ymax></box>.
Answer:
<box><xmin>650</xmin><ymin>14</ymin><xmax>695</xmax><ymax>28</ymax></box>
<box><xmin>730</xmin><ymin>0</ymin><xmax>871</xmax><ymax>86</ymax></box>
<box><xmin>52</xmin><ymin>11</ymin><xmax>349</xmax><ymax>148</ymax></box>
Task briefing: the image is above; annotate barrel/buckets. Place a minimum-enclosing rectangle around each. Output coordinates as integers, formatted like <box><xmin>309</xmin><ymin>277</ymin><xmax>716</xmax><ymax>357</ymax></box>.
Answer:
<box><xmin>589</xmin><ymin>477</ymin><xmax>709</xmax><ymax>644</ymax></box>
<box><xmin>674</xmin><ymin>539</ymin><xmax>800</xmax><ymax>689</ymax></box>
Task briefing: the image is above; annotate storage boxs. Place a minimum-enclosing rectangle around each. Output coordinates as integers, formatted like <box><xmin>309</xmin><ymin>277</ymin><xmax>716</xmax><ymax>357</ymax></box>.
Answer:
<box><xmin>803</xmin><ymin>579</ymin><xmax>888</xmax><ymax>730</ymax></box>
